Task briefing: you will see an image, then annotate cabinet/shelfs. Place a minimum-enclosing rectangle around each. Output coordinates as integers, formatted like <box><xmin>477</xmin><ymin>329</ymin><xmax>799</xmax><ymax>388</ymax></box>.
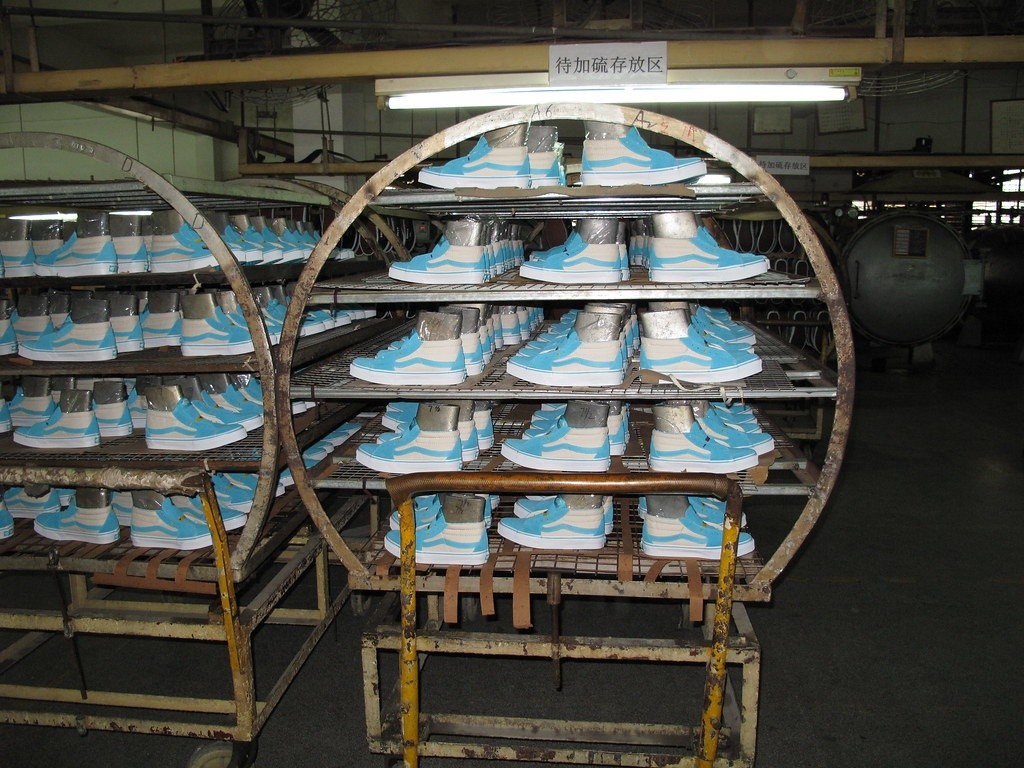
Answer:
<box><xmin>0</xmin><ymin>102</ymin><xmax>857</xmax><ymax>768</ymax></box>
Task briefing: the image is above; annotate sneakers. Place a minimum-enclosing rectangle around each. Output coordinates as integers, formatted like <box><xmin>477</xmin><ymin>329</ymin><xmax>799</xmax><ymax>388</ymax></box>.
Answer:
<box><xmin>579</xmin><ymin>126</ymin><xmax>707</xmax><ymax>187</ymax></box>
<box><xmin>0</xmin><ymin>222</ymin><xmax>774</xmax><ymax>565</ymax></box>
<box><xmin>418</xmin><ymin>134</ymin><xmax>567</xmax><ymax>190</ymax></box>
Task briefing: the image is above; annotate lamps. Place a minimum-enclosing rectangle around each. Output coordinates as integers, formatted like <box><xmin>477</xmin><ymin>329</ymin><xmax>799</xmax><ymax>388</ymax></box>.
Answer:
<box><xmin>373</xmin><ymin>65</ymin><xmax>864</xmax><ymax>111</ymax></box>
